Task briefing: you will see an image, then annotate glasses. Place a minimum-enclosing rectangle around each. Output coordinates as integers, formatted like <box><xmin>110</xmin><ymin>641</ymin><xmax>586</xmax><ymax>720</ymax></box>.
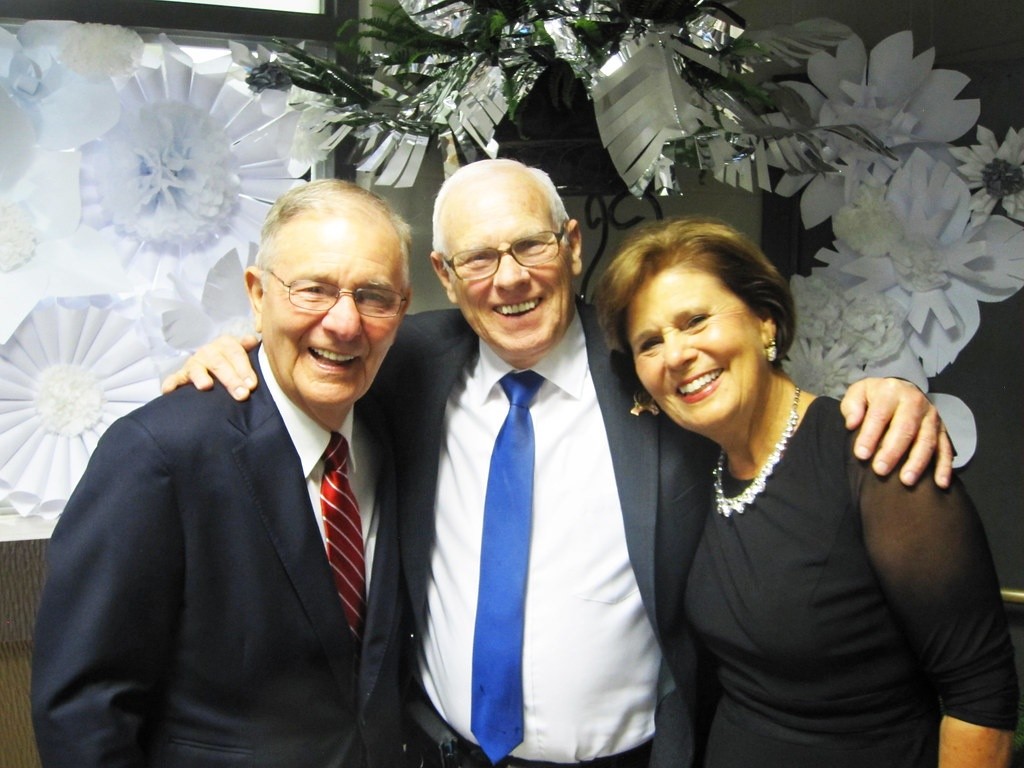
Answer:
<box><xmin>440</xmin><ymin>218</ymin><xmax>568</xmax><ymax>282</ymax></box>
<box><xmin>264</xmin><ymin>268</ymin><xmax>407</xmax><ymax>318</ymax></box>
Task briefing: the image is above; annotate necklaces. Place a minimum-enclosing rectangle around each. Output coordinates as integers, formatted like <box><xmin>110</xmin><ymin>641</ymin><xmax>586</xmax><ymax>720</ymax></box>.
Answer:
<box><xmin>711</xmin><ymin>385</ymin><xmax>801</xmax><ymax>517</ymax></box>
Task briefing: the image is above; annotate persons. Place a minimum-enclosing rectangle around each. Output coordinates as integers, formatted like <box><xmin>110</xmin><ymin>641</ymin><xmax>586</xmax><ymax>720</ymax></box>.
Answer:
<box><xmin>161</xmin><ymin>160</ymin><xmax>955</xmax><ymax>768</ymax></box>
<box><xmin>30</xmin><ymin>179</ymin><xmax>413</xmax><ymax>767</ymax></box>
<box><xmin>598</xmin><ymin>215</ymin><xmax>1020</xmax><ymax>767</ymax></box>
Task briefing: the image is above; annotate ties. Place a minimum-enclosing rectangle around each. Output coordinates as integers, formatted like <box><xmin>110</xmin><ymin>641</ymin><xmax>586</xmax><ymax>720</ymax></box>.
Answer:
<box><xmin>319</xmin><ymin>430</ymin><xmax>367</xmax><ymax>652</ymax></box>
<box><xmin>471</xmin><ymin>369</ymin><xmax>547</xmax><ymax>766</ymax></box>
